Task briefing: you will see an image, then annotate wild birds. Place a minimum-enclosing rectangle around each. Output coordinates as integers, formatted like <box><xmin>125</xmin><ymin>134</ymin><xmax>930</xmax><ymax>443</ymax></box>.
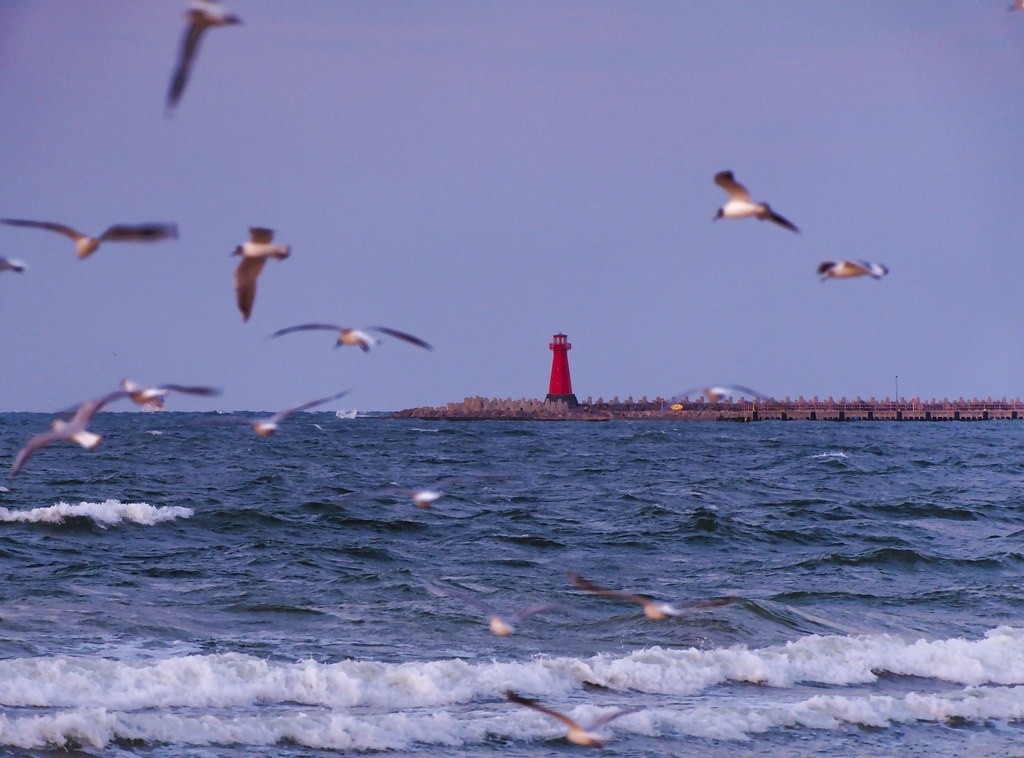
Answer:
<box><xmin>659</xmin><ymin>381</ymin><xmax>783</xmax><ymax>413</ymax></box>
<box><xmin>164</xmin><ymin>0</ymin><xmax>245</xmax><ymax>110</ymax></box>
<box><xmin>815</xmin><ymin>259</ymin><xmax>889</xmax><ymax>281</ymax></box>
<box><xmin>228</xmin><ymin>224</ymin><xmax>292</xmax><ymax>324</ymax></box>
<box><xmin>332</xmin><ymin>472</ymin><xmax>524</xmax><ymax>509</ymax></box>
<box><xmin>447</xmin><ymin>566</ymin><xmax>750</xmax><ymax>639</ymax></box>
<box><xmin>270</xmin><ymin>322</ymin><xmax>435</xmax><ymax>354</ymax></box>
<box><xmin>505</xmin><ymin>688</ymin><xmax>642</xmax><ymax>750</ymax></box>
<box><xmin>0</xmin><ymin>217</ymin><xmax>180</xmax><ymax>261</ymax></box>
<box><xmin>7</xmin><ymin>377</ymin><xmax>354</xmax><ymax>484</ymax></box>
<box><xmin>711</xmin><ymin>168</ymin><xmax>801</xmax><ymax>235</ymax></box>
